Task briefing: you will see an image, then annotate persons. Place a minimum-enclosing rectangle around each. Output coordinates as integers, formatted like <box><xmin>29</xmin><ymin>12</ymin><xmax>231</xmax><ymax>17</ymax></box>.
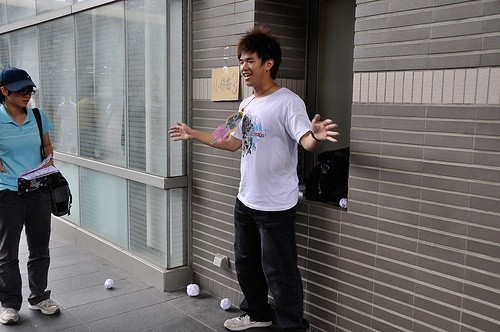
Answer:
<box><xmin>0</xmin><ymin>67</ymin><xmax>60</xmax><ymax>324</ymax></box>
<box><xmin>28</xmin><ymin>84</ymin><xmax>121</xmax><ymax>157</ymax></box>
<box><xmin>171</xmin><ymin>27</ymin><xmax>338</xmax><ymax>332</ymax></box>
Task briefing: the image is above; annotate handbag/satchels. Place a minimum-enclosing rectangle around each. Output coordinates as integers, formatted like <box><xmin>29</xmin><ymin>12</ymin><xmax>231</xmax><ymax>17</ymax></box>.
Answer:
<box><xmin>48</xmin><ymin>169</ymin><xmax>72</xmax><ymax>217</ymax></box>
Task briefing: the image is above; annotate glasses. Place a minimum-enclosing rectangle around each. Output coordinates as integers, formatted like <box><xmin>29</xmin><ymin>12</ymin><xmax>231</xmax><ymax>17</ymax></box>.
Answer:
<box><xmin>19</xmin><ymin>89</ymin><xmax>35</xmax><ymax>95</ymax></box>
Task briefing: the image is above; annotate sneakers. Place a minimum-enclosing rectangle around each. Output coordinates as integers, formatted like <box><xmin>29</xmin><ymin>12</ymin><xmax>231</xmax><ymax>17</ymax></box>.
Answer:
<box><xmin>0</xmin><ymin>302</ymin><xmax>18</xmax><ymax>324</ymax></box>
<box><xmin>224</xmin><ymin>314</ymin><xmax>272</xmax><ymax>331</ymax></box>
<box><xmin>29</xmin><ymin>298</ymin><xmax>60</xmax><ymax>315</ymax></box>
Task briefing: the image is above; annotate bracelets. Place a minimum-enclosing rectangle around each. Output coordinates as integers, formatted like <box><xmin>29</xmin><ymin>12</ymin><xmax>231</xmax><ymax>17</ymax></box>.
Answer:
<box><xmin>311</xmin><ymin>131</ymin><xmax>321</xmax><ymax>142</ymax></box>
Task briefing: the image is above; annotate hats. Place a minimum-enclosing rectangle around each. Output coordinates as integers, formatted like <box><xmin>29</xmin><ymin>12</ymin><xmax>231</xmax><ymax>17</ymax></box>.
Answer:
<box><xmin>0</xmin><ymin>66</ymin><xmax>36</xmax><ymax>91</ymax></box>
